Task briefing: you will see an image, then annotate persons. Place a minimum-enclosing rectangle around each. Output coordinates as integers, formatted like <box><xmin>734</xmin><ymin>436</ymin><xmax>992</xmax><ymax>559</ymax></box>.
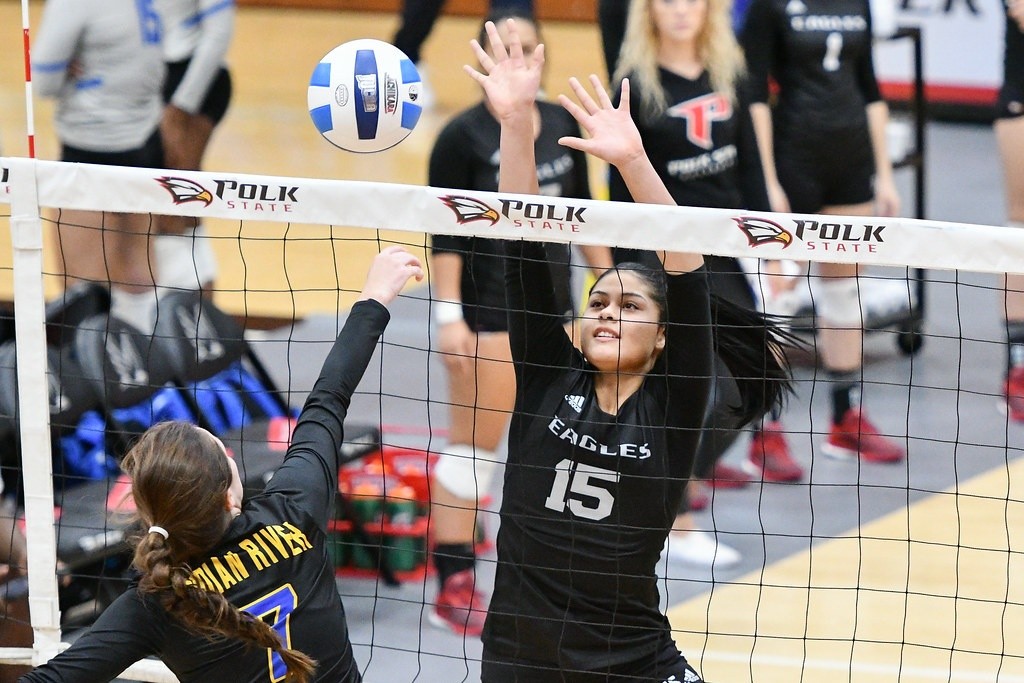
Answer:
<box><xmin>30</xmin><ymin>0</ymin><xmax>167</xmax><ymax>335</ymax></box>
<box><xmin>610</xmin><ymin>0</ymin><xmax>753</xmax><ymax>508</ymax></box>
<box><xmin>159</xmin><ymin>0</ymin><xmax>243</xmax><ymax>301</ymax></box>
<box><xmin>21</xmin><ymin>247</ymin><xmax>425</xmax><ymax>681</ymax></box>
<box><xmin>994</xmin><ymin>0</ymin><xmax>1024</xmax><ymax>422</ymax></box>
<box><xmin>429</xmin><ymin>21</ymin><xmax>611</xmax><ymax>633</ymax></box>
<box><xmin>752</xmin><ymin>0</ymin><xmax>900</xmax><ymax>482</ymax></box>
<box><xmin>392</xmin><ymin>0</ymin><xmax>443</xmax><ymax>108</ymax></box>
<box><xmin>461</xmin><ymin>16</ymin><xmax>800</xmax><ymax>683</ymax></box>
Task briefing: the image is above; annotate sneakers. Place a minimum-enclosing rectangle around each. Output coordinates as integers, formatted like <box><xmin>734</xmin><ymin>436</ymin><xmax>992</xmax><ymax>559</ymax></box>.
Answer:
<box><xmin>749</xmin><ymin>422</ymin><xmax>802</xmax><ymax>485</ymax></box>
<box><xmin>686</xmin><ymin>478</ymin><xmax>708</xmax><ymax>511</ymax></box>
<box><xmin>706</xmin><ymin>466</ymin><xmax>753</xmax><ymax>488</ymax></box>
<box><xmin>428</xmin><ymin>570</ymin><xmax>487</xmax><ymax>635</ymax></box>
<box><xmin>998</xmin><ymin>368</ymin><xmax>1024</xmax><ymax>422</ymax></box>
<box><xmin>658</xmin><ymin>528</ymin><xmax>741</xmax><ymax>569</ymax></box>
<box><xmin>820</xmin><ymin>408</ymin><xmax>905</xmax><ymax>464</ymax></box>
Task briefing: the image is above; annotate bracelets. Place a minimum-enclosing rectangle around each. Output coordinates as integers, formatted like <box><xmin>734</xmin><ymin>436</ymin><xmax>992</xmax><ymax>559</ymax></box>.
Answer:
<box><xmin>435</xmin><ymin>301</ymin><xmax>464</xmax><ymax>323</ymax></box>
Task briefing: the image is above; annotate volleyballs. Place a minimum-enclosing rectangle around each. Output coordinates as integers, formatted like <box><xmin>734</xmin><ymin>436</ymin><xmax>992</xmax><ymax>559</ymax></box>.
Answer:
<box><xmin>306</xmin><ymin>36</ymin><xmax>425</xmax><ymax>153</ymax></box>
